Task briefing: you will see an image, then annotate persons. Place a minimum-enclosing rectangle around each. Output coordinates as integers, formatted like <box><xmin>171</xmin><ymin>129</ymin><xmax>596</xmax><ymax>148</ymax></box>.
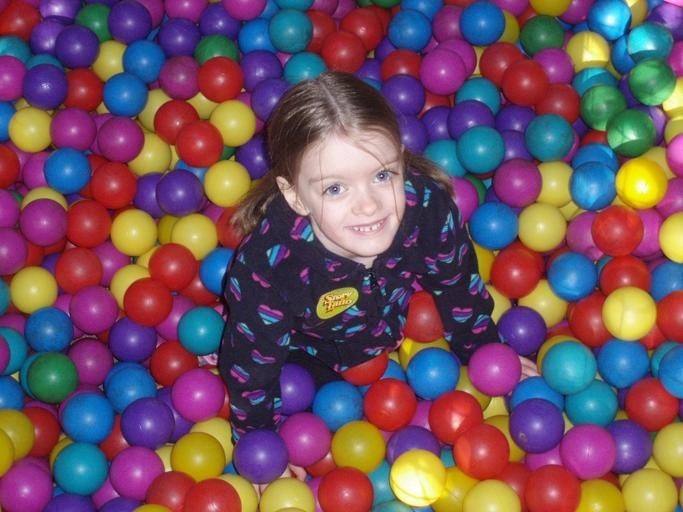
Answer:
<box><xmin>200</xmin><ymin>70</ymin><xmax>543</xmax><ymax>440</ymax></box>
